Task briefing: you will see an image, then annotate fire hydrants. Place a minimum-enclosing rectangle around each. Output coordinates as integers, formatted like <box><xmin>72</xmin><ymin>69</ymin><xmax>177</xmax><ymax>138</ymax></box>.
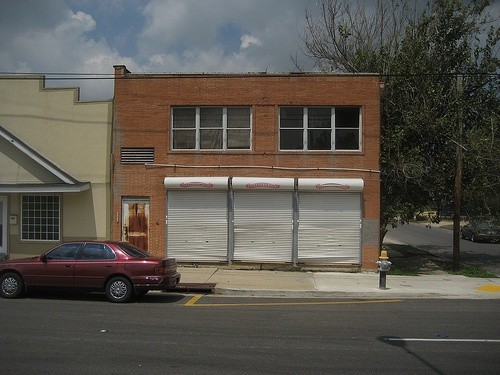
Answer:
<box><xmin>373</xmin><ymin>250</ymin><xmax>392</xmax><ymax>289</ymax></box>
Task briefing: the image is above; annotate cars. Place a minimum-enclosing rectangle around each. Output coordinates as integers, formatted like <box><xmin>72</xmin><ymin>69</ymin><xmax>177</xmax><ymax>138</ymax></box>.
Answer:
<box><xmin>461</xmin><ymin>219</ymin><xmax>499</xmax><ymax>242</ymax></box>
<box><xmin>0</xmin><ymin>241</ymin><xmax>181</xmax><ymax>304</ymax></box>
